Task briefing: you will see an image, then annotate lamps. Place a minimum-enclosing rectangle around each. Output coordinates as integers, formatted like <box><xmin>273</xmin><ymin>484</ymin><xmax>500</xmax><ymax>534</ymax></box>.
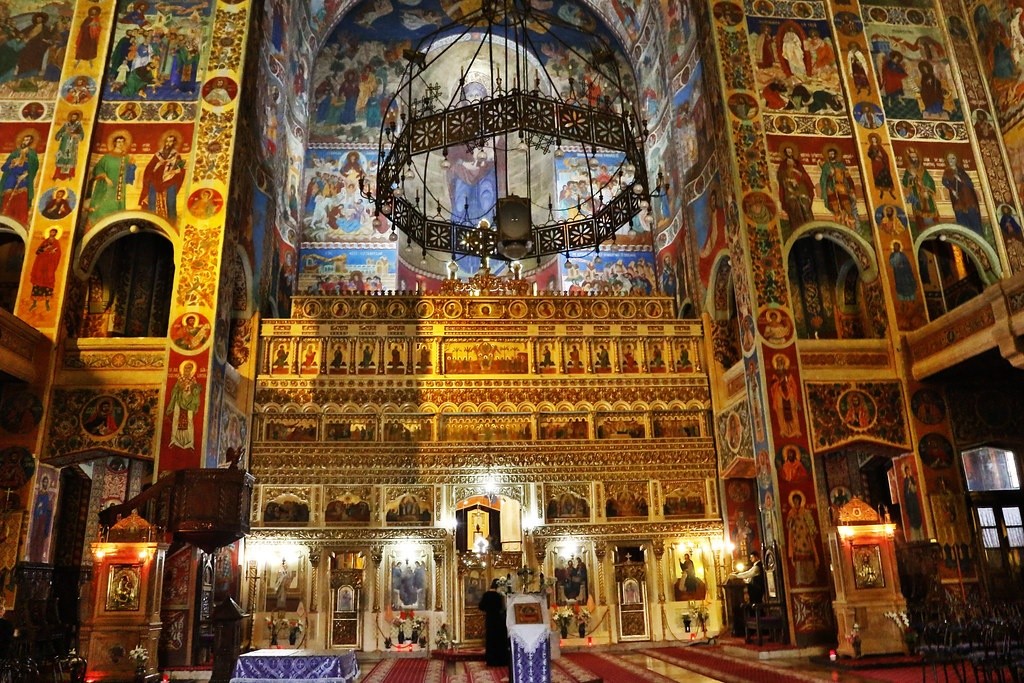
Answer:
<box><xmin>358</xmin><ymin>64</ymin><xmax>670</xmax><ymax>276</ymax></box>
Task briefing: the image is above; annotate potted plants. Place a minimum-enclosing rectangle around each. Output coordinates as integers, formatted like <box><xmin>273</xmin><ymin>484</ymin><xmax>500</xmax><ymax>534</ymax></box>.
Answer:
<box><xmin>384</xmin><ymin>637</ymin><xmax>392</xmax><ymax>653</ymax></box>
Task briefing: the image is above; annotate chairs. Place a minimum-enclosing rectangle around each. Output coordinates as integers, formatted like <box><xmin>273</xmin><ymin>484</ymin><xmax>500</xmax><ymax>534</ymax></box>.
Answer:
<box><xmin>194</xmin><ymin>553</ymin><xmax>218</xmax><ymax>665</ymax></box>
<box><xmin>740</xmin><ymin>540</ymin><xmax>783</xmax><ymax>644</ymax></box>
<box><xmin>918</xmin><ymin>599</ymin><xmax>1024</xmax><ymax>683</ymax></box>
<box><xmin>0</xmin><ymin>654</ymin><xmax>88</xmax><ymax>683</ymax></box>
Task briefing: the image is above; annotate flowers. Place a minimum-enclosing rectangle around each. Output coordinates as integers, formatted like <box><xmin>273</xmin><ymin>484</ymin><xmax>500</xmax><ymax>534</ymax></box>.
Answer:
<box><xmin>409</xmin><ymin>610</ymin><xmax>426</xmax><ymax>631</ymax></box>
<box><xmin>576</xmin><ymin>608</ymin><xmax>591</xmax><ymax>624</ymax></box>
<box><xmin>886</xmin><ymin>611</ymin><xmax>914</xmax><ymax>642</ymax></box>
<box><xmin>129</xmin><ymin>645</ymin><xmax>149</xmax><ymax>666</ymax></box>
<box><xmin>265</xmin><ymin>617</ymin><xmax>304</xmax><ymax>634</ymax></box>
<box><xmin>845</xmin><ymin>630</ymin><xmax>860</xmax><ymax>643</ymax></box>
<box><xmin>393</xmin><ymin>611</ymin><xmax>406</xmax><ymax>631</ymax></box>
<box><xmin>553</xmin><ymin>605</ymin><xmax>572</xmax><ymax>625</ymax></box>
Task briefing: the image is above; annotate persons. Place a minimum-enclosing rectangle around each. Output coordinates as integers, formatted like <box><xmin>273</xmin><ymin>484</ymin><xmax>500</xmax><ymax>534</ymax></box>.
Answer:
<box><xmin>730</xmin><ymin>551</ymin><xmax>765</xmax><ymax>605</ymax></box>
<box><xmin>478</xmin><ymin>579</ymin><xmax>508</xmax><ymax>667</ymax></box>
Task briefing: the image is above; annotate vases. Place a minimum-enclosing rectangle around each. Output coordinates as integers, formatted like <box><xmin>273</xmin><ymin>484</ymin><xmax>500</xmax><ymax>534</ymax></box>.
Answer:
<box><xmin>579</xmin><ymin>624</ymin><xmax>585</xmax><ymax>638</ymax></box>
<box><xmin>561</xmin><ymin>625</ymin><xmax>568</xmax><ymax>639</ymax></box>
<box><xmin>289</xmin><ymin>633</ymin><xmax>297</xmax><ymax>645</ymax></box>
<box><xmin>399</xmin><ymin>632</ymin><xmax>404</xmax><ymax>644</ymax></box>
<box><xmin>411</xmin><ymin>631</ymin><xmax>418</xmax><ymax>643</ymax></box>
<box><xmin>908</xmin><ymin>641</ymin><xmax>916</xmax><ymax>657</ymax></box>
<box><xmin>136</xmin><ymin>666</ymin><xmax>147</xmax><ymax>683</ymax></box>
<box><xmin>853</xmin><ymin>643</ymin><xmax>861</xmax><ymax>659</ymax></box>
<box><xmin>271</xmin><ymin>634</ymin><xmax>278</xmax><ymax>645</ymax></box>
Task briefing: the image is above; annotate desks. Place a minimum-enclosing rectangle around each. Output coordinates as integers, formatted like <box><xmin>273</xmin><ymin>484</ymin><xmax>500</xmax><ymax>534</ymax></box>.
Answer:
<box><xmin>509</xmin><ymin>624</ymin><xmax>551</xmax><ymax>683</ymax></box>
<box><xmin>233</xmin><ymin>649</ymin><xmax>361</xmax><ymax>683</ymax></box>
